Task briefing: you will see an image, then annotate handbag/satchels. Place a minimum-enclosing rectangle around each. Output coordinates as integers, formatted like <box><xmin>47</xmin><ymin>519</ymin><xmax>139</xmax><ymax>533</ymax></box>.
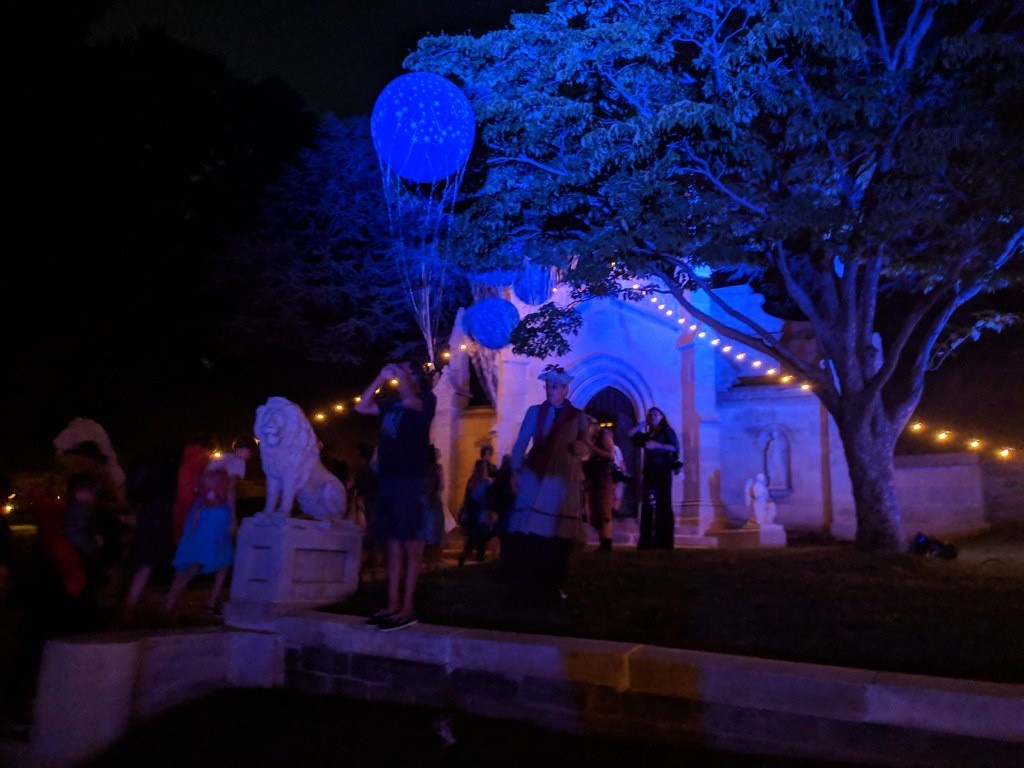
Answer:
<box><xmin>663</xmin><ymin>426</ymin><xmax>682</xmax><ymax>470</ymax></box>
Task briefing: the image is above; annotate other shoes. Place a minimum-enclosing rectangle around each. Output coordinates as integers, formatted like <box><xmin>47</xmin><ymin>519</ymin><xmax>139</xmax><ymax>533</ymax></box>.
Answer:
<box><xmin>376</xmin><ymin>614</ymin><xmax>418</xmax><ymax>632</ymax></box>
<box><xmin>365</xmin><ymin>612</ymin><xmax>400</xmax><ymax>627</ymax></box>
<box><xmin>206</xmin><ymin>605</ymin><xmax>220</xmax><ymax>615</ymax></box>
<box><xmin>551</xmin><ymin>589</ymin><xmax>567</xmax><ymax>601</ymax></box>
<box><xmin>595</xmin><ymin>537</ymin><xmax>613</xmax><ymax>551</ymax></box>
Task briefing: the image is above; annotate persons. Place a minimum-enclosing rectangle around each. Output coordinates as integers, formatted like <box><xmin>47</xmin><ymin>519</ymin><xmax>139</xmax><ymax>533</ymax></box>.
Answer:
<box><xmin>0</xmin><ymin>420</ymin><xmax>135</xmax><ymax>714</ymax></box>
<box><xmin>766</xmin><ymin>432</ymin><xmax>786</xmax><ymax>488</ymax></box>
<box><xmin>626</xmin><ymin>407</ymin><xmax>679</xmax><ymax>549</ymax></box>
<box><xmin>162</xmin><ymin>442</ymin><xmax>253</xmax><ymax>617</ymax></box>
<box><xmin>322</xmin><ymin>358</ymin><xmax>446</xmax><ymax>631</ymax></box>
<box><xmin>751</xmin><ymin>474</ymin><xmax>776</xmax><ymax>524</ymax></box>
<box><xmin>460</xmin><ymin>369</ymin><xmax>624</xmax><ymax>603</ymax></box>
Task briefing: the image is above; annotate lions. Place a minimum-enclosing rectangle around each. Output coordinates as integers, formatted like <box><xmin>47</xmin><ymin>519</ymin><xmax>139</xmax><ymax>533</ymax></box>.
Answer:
<box><xmin>253</xmin><ymin>396</ymin><xmax>348</xmax><ymax>520</ymax></box>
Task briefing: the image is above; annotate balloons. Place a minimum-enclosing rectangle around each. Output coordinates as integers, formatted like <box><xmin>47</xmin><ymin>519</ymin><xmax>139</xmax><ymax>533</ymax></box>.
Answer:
<box><xmin>372</xmin><ymin>73</ymin><xmax>476</xmax><ymax>184</ymax></box>
